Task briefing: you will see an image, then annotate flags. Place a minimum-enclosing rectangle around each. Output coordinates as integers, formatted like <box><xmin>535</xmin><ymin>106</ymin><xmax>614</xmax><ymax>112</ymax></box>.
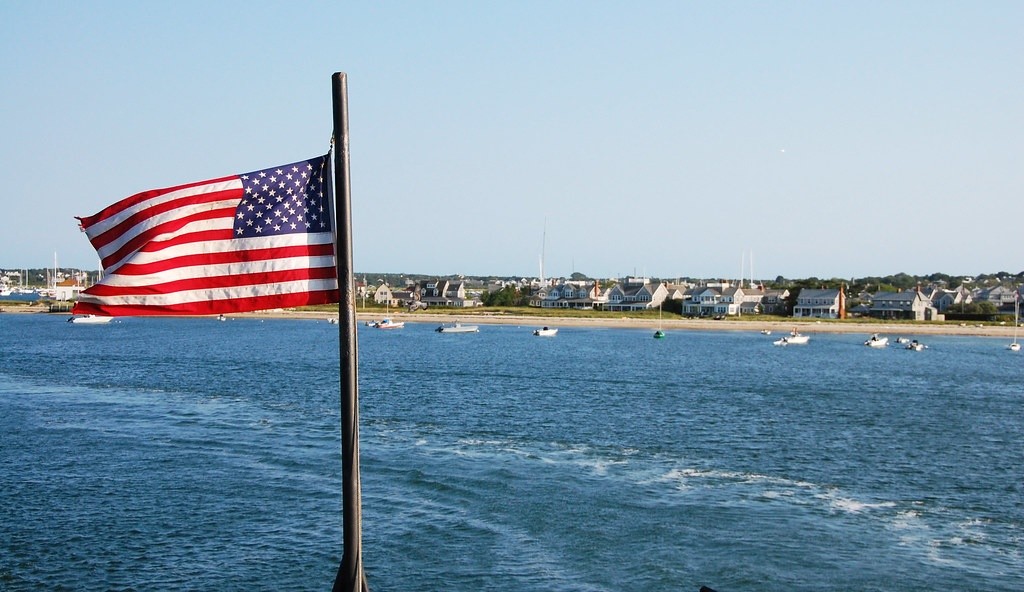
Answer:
<box><xmin>69</xmin><ymin>153</ymin><xmax>340</xmax><ymax>317</ymax></box>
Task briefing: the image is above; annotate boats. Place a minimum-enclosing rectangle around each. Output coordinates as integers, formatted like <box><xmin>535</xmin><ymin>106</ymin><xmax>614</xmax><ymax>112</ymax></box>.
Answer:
<box><xmin>435</xmin><ymin>325</ymin><xmax>478</xmax><ymax>333</ymax></box>
<box><xmin>865</xmin><ymin>333</ymin><xmax>888</xmax><ymax>348</ymax></box>
<box><xmin>1010</xmin><ymin>343</ymin><xmax>1021</xmax><ymax>351</ymax></box>
<box><xmin>654</xmin><ymin>330</ymin><xmax>665</xmax><ymax>339</ymax></box>
<box><xmin>897</xmin><ymin>336</ymin><xmax>929</xmax><ymax>352</ymax></box>
<box><xmin>327</xmin><ymin>317</ymin><xmax>339</xmax><ymax>324</ymax></box>
<box><xmin>68</xmin><ymin>314</ymin><xmax>114</xmax><ymax>325</ymax></box>
<box><xmin>533</xmin><ymin>327</ymin><xmax>558</xmax><ymax>336</ymax></box>
<box><xmin>365</xmin><ymin>319</ymin><xmax>405</xmax><ymax>330</ymax></box>
<box><xmin>760</xmin><ymin>329</ymin><xmax>771</xmax><ymax>335</ymax></box>
<box><xmin>773</xmin><ymin>327</ymin><xmax>810</xmax><ymax>347</ymax></box>
<box><xmin>216</xmin><ymin>314</ymin><xmax>226</xmax><ymax>321</ymax></box>
